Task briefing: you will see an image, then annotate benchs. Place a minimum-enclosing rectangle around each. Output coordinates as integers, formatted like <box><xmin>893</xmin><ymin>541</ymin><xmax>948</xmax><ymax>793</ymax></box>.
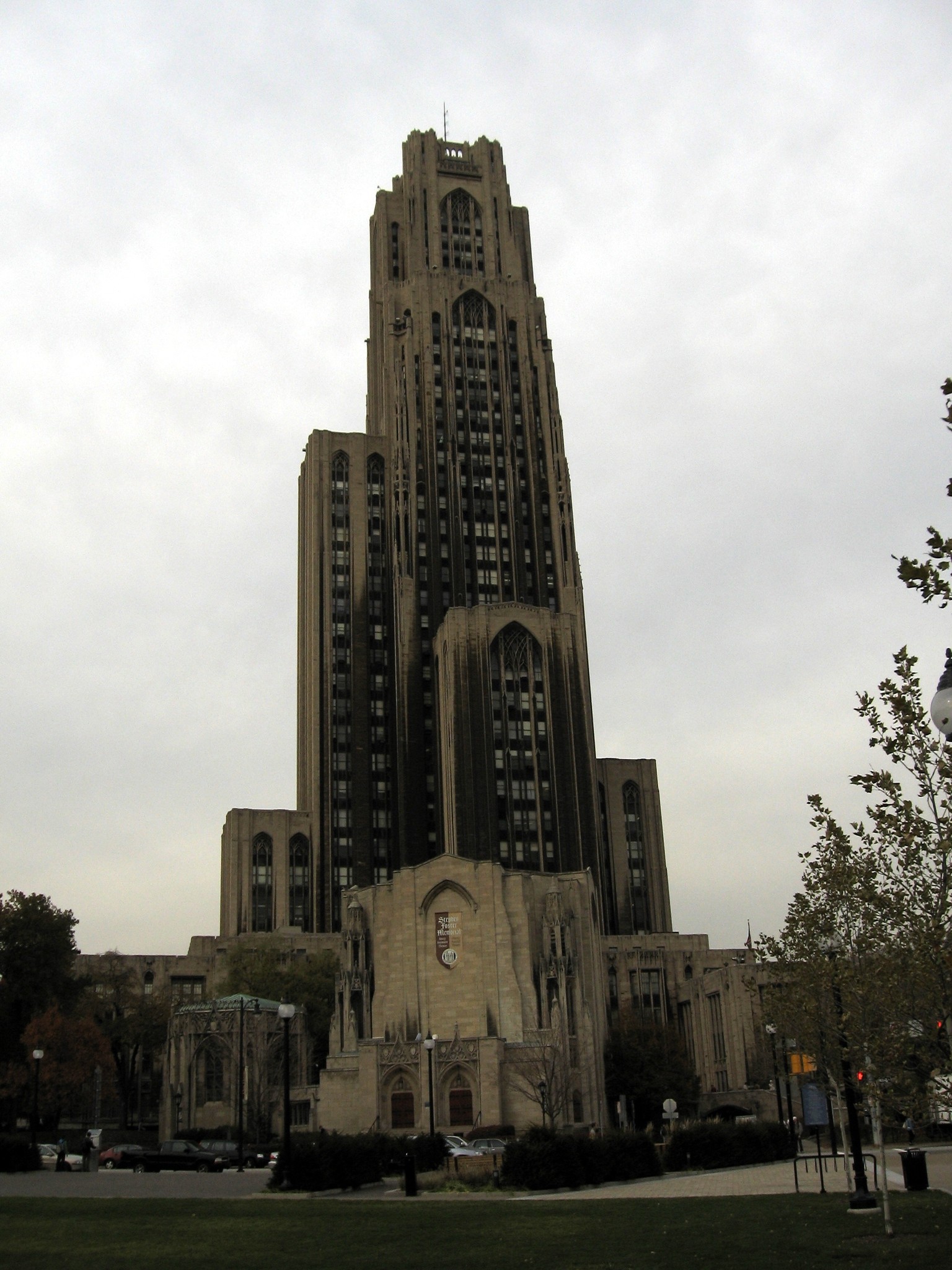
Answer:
<box><xmin>444</xmin><ymin>1154</ymin><xmax>502</xmax><ymax>1176</ymax></box>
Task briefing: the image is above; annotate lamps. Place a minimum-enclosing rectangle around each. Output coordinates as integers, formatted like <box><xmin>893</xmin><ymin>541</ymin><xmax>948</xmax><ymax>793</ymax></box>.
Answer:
<box><xmin>492</xmin><ymin>1169</ymin><xmax>500</xmax><ymax>1178</ymax></box>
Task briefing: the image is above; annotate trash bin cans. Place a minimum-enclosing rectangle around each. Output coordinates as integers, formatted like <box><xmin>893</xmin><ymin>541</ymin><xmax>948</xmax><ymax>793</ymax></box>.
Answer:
<box><xmin>898</xmin><ymin>1146</ymin><xmax>929</xmax><ymax>1190</ymax></box>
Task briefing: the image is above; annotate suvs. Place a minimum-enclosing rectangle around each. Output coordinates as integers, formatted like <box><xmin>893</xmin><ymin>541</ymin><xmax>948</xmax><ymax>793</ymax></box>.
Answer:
<box><xmin>198</xmin><ymin>1140</ymin><xmax>269</xmax><ymax>1170</ymax></box>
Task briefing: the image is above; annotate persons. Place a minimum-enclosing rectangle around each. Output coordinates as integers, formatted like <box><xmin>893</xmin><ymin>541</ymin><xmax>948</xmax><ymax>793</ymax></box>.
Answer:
<box><xmin>769</xmin><ymin>1080</ymin><xmax>775</xmax><ymax>1090</ymax></box>
<box><xmin>778</xmin><ymin>1117</ymin><xmax>804</xmax><ymax>1153</ymax></box>
<box><xmin>905</xmin><ymin>1114</ymin><xmax>916</xmax><ymax>1146</ymax></box>
<box><xmin>711</xmin><ymin>1084</ymin><xmax>716</xmax><ymax>1092</ymax></box>
<box><xmin>82</xmin><ymin>1130</ymin><xmax>98</xmax><ymax>1171</ymax></box>
<box><xmin>744</xmin><ymin>1082</ymin><xmax>748</xmax><ymax>1090</ymax></box>
<box><xmin>55</xmin><ymin>1136</ymin><xmax>68</xmax><ymax>1170</ymax></box>
<box><xmin>589</xmin><ymin>1122</ymin><xmax>598</xmax><ymax>1141</ymax></box>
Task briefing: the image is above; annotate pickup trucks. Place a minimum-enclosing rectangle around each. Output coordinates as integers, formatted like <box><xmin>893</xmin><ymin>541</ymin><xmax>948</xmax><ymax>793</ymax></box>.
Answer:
<box><xmin>126</xmin><ymin>1138</ymin><xmax>231</xmax><ymax>1173</ymax></box>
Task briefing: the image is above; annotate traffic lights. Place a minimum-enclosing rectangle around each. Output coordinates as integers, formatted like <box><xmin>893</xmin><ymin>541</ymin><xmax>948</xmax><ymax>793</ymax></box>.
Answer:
<box><xmin>937</xmin><ymin>1019</ymin><xmax>951</xmax><ymax>1055</ymax></box>
<box><xmin>857</xmin><ymin>1071</ymin><xmax>868</xmax><ymax>1084</ymax></box>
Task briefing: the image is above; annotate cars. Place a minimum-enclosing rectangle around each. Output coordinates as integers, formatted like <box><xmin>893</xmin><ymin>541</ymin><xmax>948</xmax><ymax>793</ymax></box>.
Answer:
<box><xmin>407</xmin><ymin>1135</ymin><xmax>507</xmax><ymax>1158</ymax></box>
<box><xmin>96</xmin><ymin>1143</ymin><xmax>149</xmax><ymax>1171</ymax></box>
<box><xmin>31</xmin><ymin>1144</ymin><xmax>86</xmax><ymax>1171</ymax></box>
<box><xmin>267</xmin><ymin>1149</ymin><xmax>279</xmax><ymax>1171</ymax></box>
<box><xmin>85</xmin><ymin>1129</ymin><xmax>102</xmax><ymax>1148</ymax></box>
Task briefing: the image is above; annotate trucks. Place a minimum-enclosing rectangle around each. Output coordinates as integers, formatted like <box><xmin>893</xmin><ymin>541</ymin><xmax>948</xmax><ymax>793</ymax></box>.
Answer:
<box><xmin>926</xmin><ymin>1074</ymin><xmax>952</xmax><ymax>1135</ymax></box>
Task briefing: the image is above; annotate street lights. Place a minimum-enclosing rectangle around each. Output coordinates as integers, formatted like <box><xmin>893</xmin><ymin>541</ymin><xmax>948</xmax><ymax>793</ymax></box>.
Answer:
<box><xmin>235</xmin><ymin>996</ymin><xmax>263</xmax><ymax>1172</ymax></box>
<box><xmin>763</xmin><ymin>1021</ymin><xmax>786</xmax><ymax>1127</ymax></box>
<box><xmin>423</xmin><ymin>1029</ymin><xmax>437</xmax><ymax>1137</ymax></box>
<box><xmin>32</xmin><ymin>1042</ymin><xmax>45</xmax><ymax>1141</ymax></box>
<box><xmin>173</xmin><ymin>1092</ymin><xmax>182</xmax><ymax>1139</ymax></box>
<box><xmin>537</xmin><ymin>1080</ymin><xmax>547</xmax><ymax>1130</ymax></box>
<box><xmin>277</xmin><ymin>995</ymin><xmax>296</xmax><ymax>1140</ymax></box>
